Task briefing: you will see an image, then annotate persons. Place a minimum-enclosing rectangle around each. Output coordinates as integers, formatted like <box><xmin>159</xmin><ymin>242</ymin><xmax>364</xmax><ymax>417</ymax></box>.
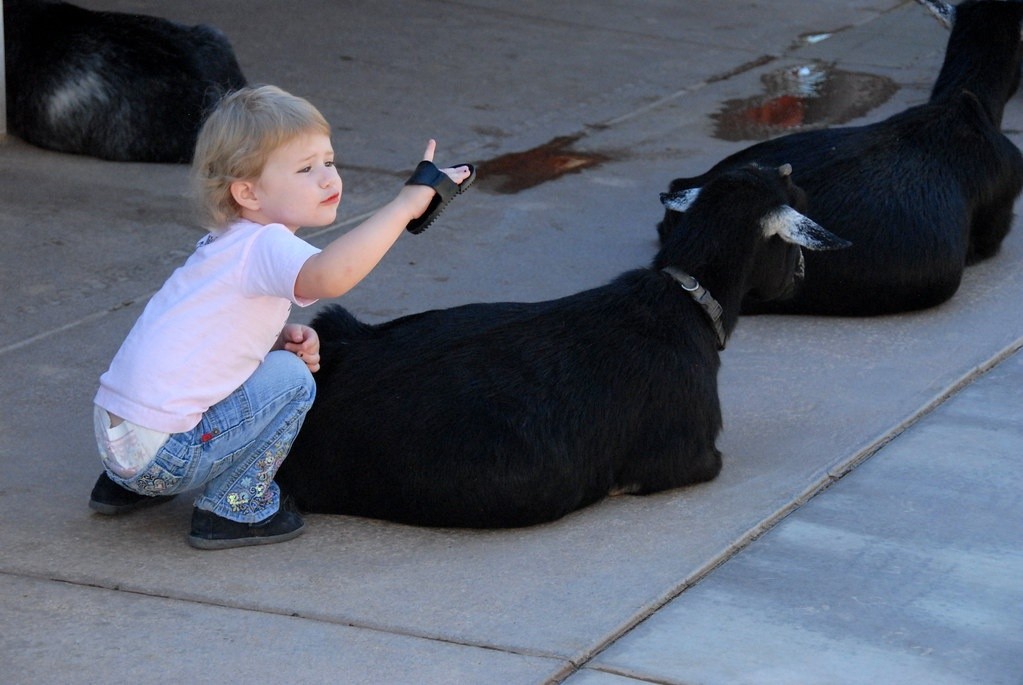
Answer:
<box><xmin>87</xmin><ymin>82</ymin><xmax>471</xmax><ymax>550</ymax></box>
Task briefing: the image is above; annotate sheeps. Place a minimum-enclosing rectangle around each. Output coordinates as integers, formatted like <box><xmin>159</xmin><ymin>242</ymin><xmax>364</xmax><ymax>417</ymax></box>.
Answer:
<box><xmin>1</xmin><ymin>0</ymin><xmax>247</xmax><ymax>161</ymax></box>
<box><xmin>274</xmin><ymin>1</ymin><xmax>1023</xmax><ymax>527</ymax></box>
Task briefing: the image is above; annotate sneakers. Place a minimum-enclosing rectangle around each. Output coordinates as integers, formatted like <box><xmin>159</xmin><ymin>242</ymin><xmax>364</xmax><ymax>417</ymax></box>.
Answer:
<box><xmin>87</xmin><ymin>470</ymin><xmax>178</xmax><ymax>515</ymax></box>
<box><xmin>189</xmin><ymin>505</ymin><xmax>305</xmax><ymax>550</ymax></box>
